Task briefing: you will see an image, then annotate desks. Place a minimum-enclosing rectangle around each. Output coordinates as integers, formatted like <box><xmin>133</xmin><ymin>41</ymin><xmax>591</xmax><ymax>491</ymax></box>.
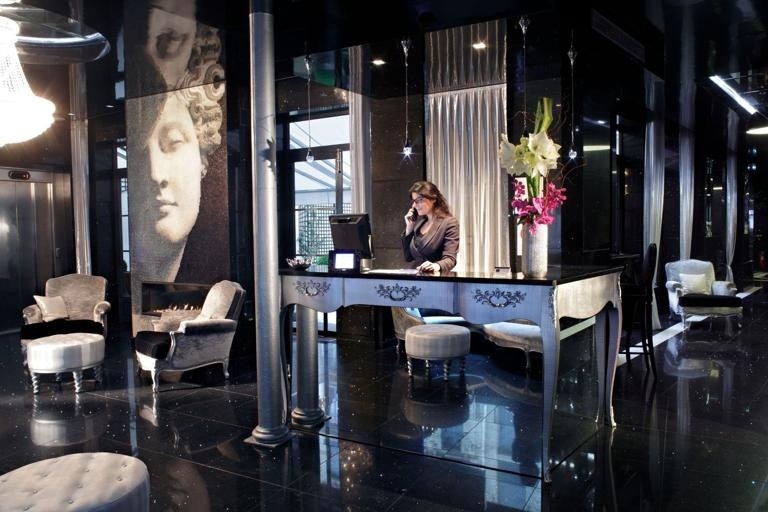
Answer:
<box><xmin>277</xmin><ymin>265</ymin><xmax>626</xmax><ymax>485</ymax></box>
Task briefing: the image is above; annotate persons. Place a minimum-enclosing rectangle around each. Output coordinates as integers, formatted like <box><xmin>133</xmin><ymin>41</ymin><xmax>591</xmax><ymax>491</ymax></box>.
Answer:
<box><xmin>124</xmin><ymin>1</ymin><xmax>225</xmax><ymax>96</ymax></box>
<box><xmin>401</xmin><ymin>182</ymin><xmax>461</xmax><ymax>274</ymax></box>
<box><xmin>127</xmin><ymin>84</ymin><xmax>223</xmax><ymax>281</ymax></box>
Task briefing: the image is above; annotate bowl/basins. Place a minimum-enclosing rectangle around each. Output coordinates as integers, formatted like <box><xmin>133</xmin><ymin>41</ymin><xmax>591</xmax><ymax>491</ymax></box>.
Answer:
<box><xmin>287</xmin><ymin>259</ymin><xmax>311</xmax><ymax>270</ymax></box>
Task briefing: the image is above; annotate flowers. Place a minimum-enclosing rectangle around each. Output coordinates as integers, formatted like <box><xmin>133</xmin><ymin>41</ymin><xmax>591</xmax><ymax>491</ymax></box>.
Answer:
<box><xmin>500</xmin><ymin>131</ymin><xmax>567</xmax><ymax>231</ymax></box>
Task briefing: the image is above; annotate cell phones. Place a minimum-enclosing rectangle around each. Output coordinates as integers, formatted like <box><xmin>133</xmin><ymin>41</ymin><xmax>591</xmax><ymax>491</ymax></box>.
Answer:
<box><xmin>410</xmin><ymin>205</ymin><xmax>418</xmax><ymax>222</ymax></box>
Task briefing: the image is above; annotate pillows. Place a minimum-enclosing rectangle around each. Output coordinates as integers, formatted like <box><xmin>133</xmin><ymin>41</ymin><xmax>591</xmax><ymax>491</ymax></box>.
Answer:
<box><xmin>33</xmin><ymin>293</ymin><xmax>69</xmax><ymax>323</ymax></box>
<box><xmin>678</xmin><ymin>272</ymin><xmax>709</xmax><ymax>295</ymax></box>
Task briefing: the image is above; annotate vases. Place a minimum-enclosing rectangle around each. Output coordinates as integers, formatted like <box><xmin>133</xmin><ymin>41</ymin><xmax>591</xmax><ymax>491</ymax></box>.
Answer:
<box><xmin>521</xmin><ymin>224</ymin><xmax>550</xmax><ymax>279</ymax></box>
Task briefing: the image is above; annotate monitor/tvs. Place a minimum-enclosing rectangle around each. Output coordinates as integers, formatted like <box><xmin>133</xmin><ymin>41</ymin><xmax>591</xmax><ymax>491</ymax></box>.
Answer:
<box><xmin>329</xmin><ymin>214</ymin><xmax>374</xmax><ymax>259</ymax></box>
<box><xmin>328</xmin><ymin>250</ymin><xmax>360</xmax><ymax>274</ymax></box>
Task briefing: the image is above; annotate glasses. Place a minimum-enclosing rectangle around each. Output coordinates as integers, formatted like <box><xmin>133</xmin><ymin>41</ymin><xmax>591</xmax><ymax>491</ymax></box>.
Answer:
<box><xmin>407</xmin><ymin>197</ymin><xmax>427</xmax><ymax>208</ymax></box>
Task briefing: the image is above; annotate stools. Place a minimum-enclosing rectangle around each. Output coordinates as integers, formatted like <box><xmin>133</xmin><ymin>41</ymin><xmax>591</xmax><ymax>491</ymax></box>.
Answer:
<box><xmin>608</xmin><ymin>252</ymin><xmax>642</xmax><ymax>285</ymax></box>
<box><xmin>0</xmin><ymin>452</ymin><xmax>150</xmax><ymax>512</ymax></box>
<box><xmin>404</xmin><ymin>323</ymin><xmax>470</xmax><ymax>403</ymax></box>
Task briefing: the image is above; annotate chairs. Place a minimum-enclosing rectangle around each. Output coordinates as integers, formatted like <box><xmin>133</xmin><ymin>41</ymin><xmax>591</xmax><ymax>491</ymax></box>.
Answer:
<box><xmin>664</xmin><ymin>258</ymin><xmax>746</xmax><ymax>343</ymax></box>
<box><xmin>134</xmin><ymin>280</ymin><xmax>247</xmax><ymax>420</ymax></box>
<box><xmin>611</xmin><ymin>243</ymin><xmax>658</xmax><ymax>380</ymax></box>
<box><xmin>22</xmin><ymin>273</ymin><xmax>110</xmax><ymax>384</ymax></box>
